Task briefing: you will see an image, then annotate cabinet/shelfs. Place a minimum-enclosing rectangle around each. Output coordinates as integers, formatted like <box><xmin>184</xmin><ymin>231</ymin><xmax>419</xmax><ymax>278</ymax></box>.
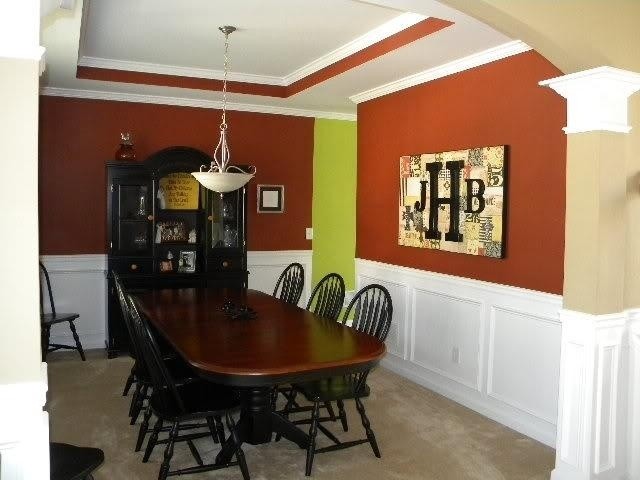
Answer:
<box><xmin>106</xmin><ymin>146</ymin><xmax>251</xmax><ymax>359</ymax></box>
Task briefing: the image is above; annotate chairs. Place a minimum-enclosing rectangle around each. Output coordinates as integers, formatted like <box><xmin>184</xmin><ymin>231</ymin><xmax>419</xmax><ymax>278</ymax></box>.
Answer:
<box><xmin>40</xmin><ymin>261</ymin><xmax>86</xmax><ymax>361</ymax></box>
<box><xmin>112</xmin><ymin>269</ymin><xmax>251</xmax><ymax>479</ymax></box>
<box><xmin>271</xmin><ymin>273</ymin><xmax>345</xmax><ymax>423</ymax></box>
<box><xmin>276</xmin><ymin>283</ymin><xmax>394</xmax><ymax>475</ymax></box>
<box><xmin>50</xmin><ymin>442</ymin><xmax>104</xmax><ymax>480</ymax></box>
<box><xmin>272</xmin><ymin>263</ymin><xmax>304</xmax><ymax>304</ymax></box>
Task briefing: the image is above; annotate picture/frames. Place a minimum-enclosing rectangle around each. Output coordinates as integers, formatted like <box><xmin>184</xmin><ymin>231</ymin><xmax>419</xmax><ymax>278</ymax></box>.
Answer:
<box><xmin>257</xmin><ymin>185</ymin><xmax>284</xmax><ymax>215</ymax></box>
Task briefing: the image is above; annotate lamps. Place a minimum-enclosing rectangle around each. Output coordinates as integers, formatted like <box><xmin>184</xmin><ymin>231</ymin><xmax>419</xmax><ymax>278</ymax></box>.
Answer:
<box><xmin>192</xmin><ymin>26</ymin><xmax>256</xmax><ymax>193</ymax></box>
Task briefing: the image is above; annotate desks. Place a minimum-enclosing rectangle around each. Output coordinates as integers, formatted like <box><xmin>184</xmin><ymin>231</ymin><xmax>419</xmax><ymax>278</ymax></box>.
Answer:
<box><xmin>128</xmin><ymin>288</ymin><xmax>385</xmax><ymax>466</ymax></box>
